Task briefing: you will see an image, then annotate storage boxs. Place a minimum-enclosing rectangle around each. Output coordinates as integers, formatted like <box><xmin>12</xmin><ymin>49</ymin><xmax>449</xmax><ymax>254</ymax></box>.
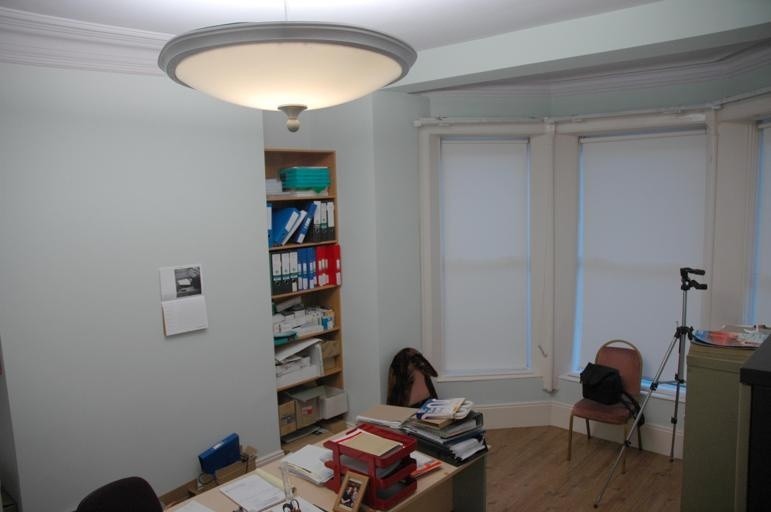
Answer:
<box><xmin>276</xmin><ymin>385</ymin><xmax>347</xmax><ymax>441</ymax></box>
<box><xmin>203</xmin><ymin>446</ymin><xmax>257</xmax><ymax>491</ymax></box>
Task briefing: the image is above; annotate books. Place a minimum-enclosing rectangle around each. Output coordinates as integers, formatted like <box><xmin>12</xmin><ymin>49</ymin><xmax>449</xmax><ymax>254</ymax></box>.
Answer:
<box><xmin>266</xmin><ymin>173</ymin><xmax>341</xmax><ymax>390</ymax></box>
<box><xmin>355</xmin><ymin>397</ymin><xmax>488</xmax><ymax>467</ymax></box>
<box><xmin>219</xmin><ymin>468</ymin><xmax>326</xmax><ymax>512</ymax></box>
<box><xmin>280</xmin><ymin>420</ymin><xmax>444</xmax><ymax>512</ymax></box>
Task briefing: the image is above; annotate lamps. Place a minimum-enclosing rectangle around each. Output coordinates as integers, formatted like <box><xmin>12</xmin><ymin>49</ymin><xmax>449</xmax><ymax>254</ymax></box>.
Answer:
<box><xmin>155</xmin><ymin>21</ymin><xmax>417</xmax><ymax>134</ymax></box>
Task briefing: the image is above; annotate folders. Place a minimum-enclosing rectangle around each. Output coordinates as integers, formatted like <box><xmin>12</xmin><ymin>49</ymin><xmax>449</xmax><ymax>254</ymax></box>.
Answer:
<box><xmin>267</xmin><ymin>201</ymin><xmax>341</xmax><ymax>296</ymax></box>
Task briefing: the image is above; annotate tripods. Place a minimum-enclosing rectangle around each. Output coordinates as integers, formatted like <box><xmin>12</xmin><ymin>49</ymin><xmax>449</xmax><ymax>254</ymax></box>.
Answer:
<box><xmin>593</xmin><ymin>268</ymin><xmax>707</xmax><ymax>509</ymax></box>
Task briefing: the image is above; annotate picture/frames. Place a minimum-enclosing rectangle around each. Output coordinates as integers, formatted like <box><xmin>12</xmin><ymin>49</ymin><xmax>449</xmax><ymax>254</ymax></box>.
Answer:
<box><xmin>332</xmin><ymin>469</ymin><xmax>370</xmax><ymax>512</ymax></box>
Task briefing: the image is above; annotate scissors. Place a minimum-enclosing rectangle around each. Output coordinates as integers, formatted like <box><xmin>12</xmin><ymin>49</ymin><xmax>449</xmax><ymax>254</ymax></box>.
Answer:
<box><xmin>283</xmin><ymin>499</ymin><xmax>299</xmax><ymax>512</ymax></box>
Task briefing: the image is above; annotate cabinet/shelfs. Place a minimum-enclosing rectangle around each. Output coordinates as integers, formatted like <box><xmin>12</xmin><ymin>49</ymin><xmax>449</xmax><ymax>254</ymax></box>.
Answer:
<box><xmin>263</xmin><ymin>146</ymin><xmax>349</xmax><ymax>456</ymax></box>
<box><xmin>323</xmin><ymin>423</ymin><xmax>417</xmax><ymax>512</ymax></box>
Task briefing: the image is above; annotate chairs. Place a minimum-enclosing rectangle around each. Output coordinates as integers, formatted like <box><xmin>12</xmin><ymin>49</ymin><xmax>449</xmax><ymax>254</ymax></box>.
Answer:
<box><xmin>387</xmin><ymin>347</ymin><xmax>439</xmax><ymax>408</ymax></box>
<box><xmin>564</xmin><ymin>338</ymin><xmax>644</xmax><ymax>475</ymax></box>
<box><xmin>77</xmin><ymin>477</ymin><xmax>163</xmax><ymax>512</ymax></box>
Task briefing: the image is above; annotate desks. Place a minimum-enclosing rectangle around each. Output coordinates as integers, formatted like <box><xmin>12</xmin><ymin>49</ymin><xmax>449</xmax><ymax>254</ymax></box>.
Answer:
<box><xmin>163</xmin><ymin>403</ymin><xmax>491</xmax><ymax>512</ymax></box>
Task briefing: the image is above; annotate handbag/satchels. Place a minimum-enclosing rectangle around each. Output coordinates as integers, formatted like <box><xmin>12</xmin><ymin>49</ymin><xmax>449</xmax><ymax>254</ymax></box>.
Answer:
<box><xmin>579</xmin><ymin>362</ymin><xmax>646</xmax><ymax>426</ymax></box>
